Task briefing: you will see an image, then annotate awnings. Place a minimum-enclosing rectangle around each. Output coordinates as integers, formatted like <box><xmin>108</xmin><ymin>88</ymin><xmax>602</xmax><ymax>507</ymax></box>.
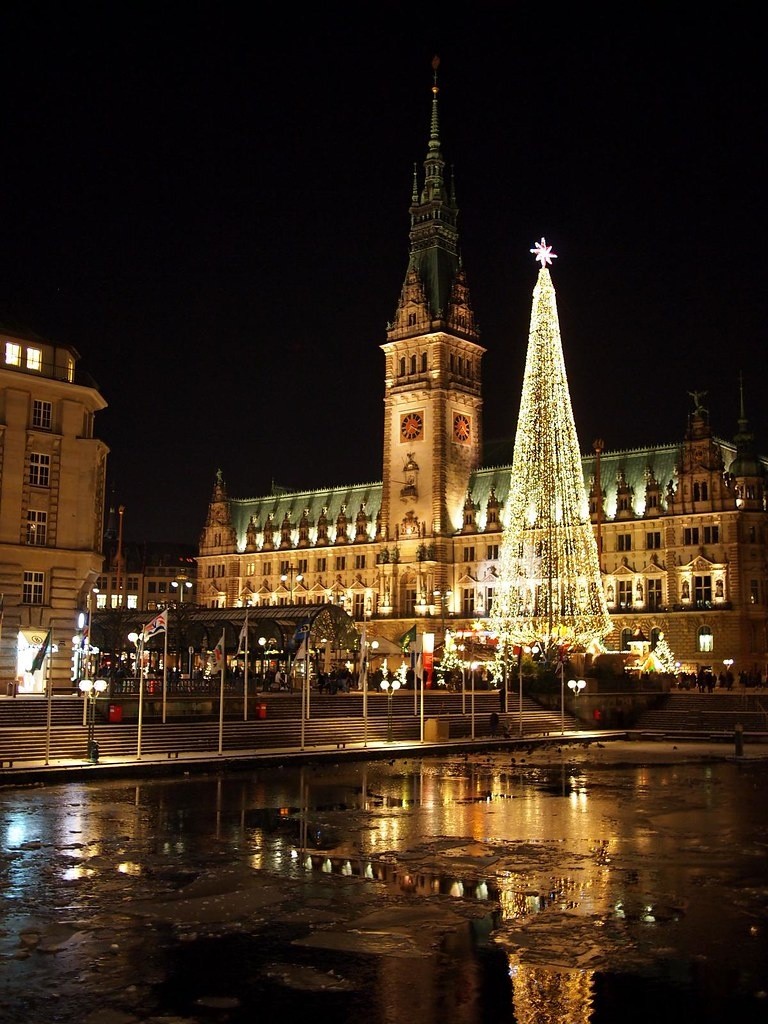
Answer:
<box><xmin>18</xmin><ymin>630</ymin><xmax>50</xmax><ymax>651</ymax></box>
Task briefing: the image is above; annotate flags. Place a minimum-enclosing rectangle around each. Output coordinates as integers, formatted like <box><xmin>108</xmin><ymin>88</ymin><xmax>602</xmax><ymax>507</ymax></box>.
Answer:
<box><xmin>292</xmin><ymin>619</ymin><xmax>311</xmax><ymax>641</ymax></box>
<box><xmin>140</xmin><ymin>609</ymin><xmax>167</xmax><ymax>641</ymax></box>
<box><xmin>213</xmin><ymin>637</ymin><xmax>223</xmax><ymax>665</ymax></box>
<box><xmin>237</xmin><ymin>619</ymin><xmax>247</xmax><ymax>654</ymax></box>
<box><xmin>82</xmin><ymin>611</ymin><xmax>89</xmax><ymax>648</ymax></box>
<box><xmin>31</xmin><ymin>630</ymin><xmax>51</xmax><ymax>673</ymax></box>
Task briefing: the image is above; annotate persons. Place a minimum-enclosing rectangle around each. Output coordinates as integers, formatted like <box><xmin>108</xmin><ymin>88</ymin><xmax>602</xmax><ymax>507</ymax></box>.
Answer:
<box><xmin>499</xmin><ymin>685</ymin><xmax>506</xmax><ymax>712</ymax></box>
<box><xmin>116</xmin><ymin>661</ymin><xmax>495</xmax><ymax>694</ymax></box>
<box><xmin>489</xmin><ymin>710</ymin><xmax>499</xmax><ymax>736</ymax></box>
<box><xmin>644</xmin><ymin>668</ymin><xmax>768</xmax><ymax>693</ymax></box>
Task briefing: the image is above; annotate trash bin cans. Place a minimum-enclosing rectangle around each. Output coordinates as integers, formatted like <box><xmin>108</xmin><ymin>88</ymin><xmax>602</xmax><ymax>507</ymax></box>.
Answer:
<box><xmin>7</xmin><ymin>681</ymin><xmax>14</xmax><ymax>696</ymax></box>
<box><xmin>108</xmin><ymin>705</ymin><xmax>123</xmax><ymax>723</ymax></box>
<box><xmin>88</xmin><ymin>740</ymin><xmax>100</xmax><ymax>762</ymax></box>
<box><xmin>424</xmin><ymin>718</ymin><xmax>450</xmax><ymax>742</ymax></box>
<box><xmin>255</xmin><ymin>701</ymin><xmax>267</xmax><ymax>719</ymax></box>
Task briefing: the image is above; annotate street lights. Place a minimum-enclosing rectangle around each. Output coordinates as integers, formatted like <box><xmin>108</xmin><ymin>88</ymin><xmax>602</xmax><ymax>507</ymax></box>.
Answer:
<box><xmin>567</xmin><ymin>679</ymin><xmax>587</xmax><ymax>730</ymax></box>
<box><xmin>380</xmin><ymin>680</ymin><xmax>402</xmax><ymax>741</ymax></box>
<box><xmin>280</xmin><ymin>562</ymin><xmax>304</xmax><ymax>601</ymax></box>
<box><xmin>171</xmin><ymin>574</ymin><xmax>194</xmax><ymax>602</ymax></box>
<box><xmin>432</xmin><ymin>582</ymin><xmax>454</xmax><ymax>632</ymax></box>
<box><xmin>79</xmin><ymin>679</ymin><xmax>107</xmax><ymax>742</ymax></box>
